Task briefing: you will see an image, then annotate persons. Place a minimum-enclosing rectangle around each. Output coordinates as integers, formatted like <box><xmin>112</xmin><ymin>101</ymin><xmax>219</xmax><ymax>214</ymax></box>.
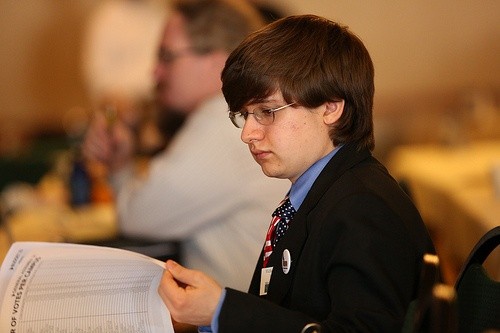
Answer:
<box><xmin>156</xmin><ymin>12</ymin><xmax>438</xmax><ymax>333</ymax></box>
<box><xmin>83</xmin><ymin>0</ymin><xmax>292</xmax><ymax>294</ymax></box>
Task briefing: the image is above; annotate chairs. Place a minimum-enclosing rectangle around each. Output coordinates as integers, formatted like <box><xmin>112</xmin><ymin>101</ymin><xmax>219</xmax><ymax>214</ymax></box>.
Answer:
<box><xmin>454</xmin><ymin>226</ymin><xmax>500</xmax><ymax>333</ymax></box>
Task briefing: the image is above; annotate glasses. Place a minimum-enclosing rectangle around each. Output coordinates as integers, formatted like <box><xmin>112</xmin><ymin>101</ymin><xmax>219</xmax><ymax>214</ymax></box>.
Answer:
<box><xmin>228</xmin><ymin>101</ymin><xmax>298</xmax><ymax>128</ymax></box>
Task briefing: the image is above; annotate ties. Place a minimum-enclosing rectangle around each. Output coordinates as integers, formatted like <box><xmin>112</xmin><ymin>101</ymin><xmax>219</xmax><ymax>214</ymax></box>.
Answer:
<box><xmin>263</xmin><ymin>192</ymin><xmax>296</xmax><ymax>268</ymax></box>
